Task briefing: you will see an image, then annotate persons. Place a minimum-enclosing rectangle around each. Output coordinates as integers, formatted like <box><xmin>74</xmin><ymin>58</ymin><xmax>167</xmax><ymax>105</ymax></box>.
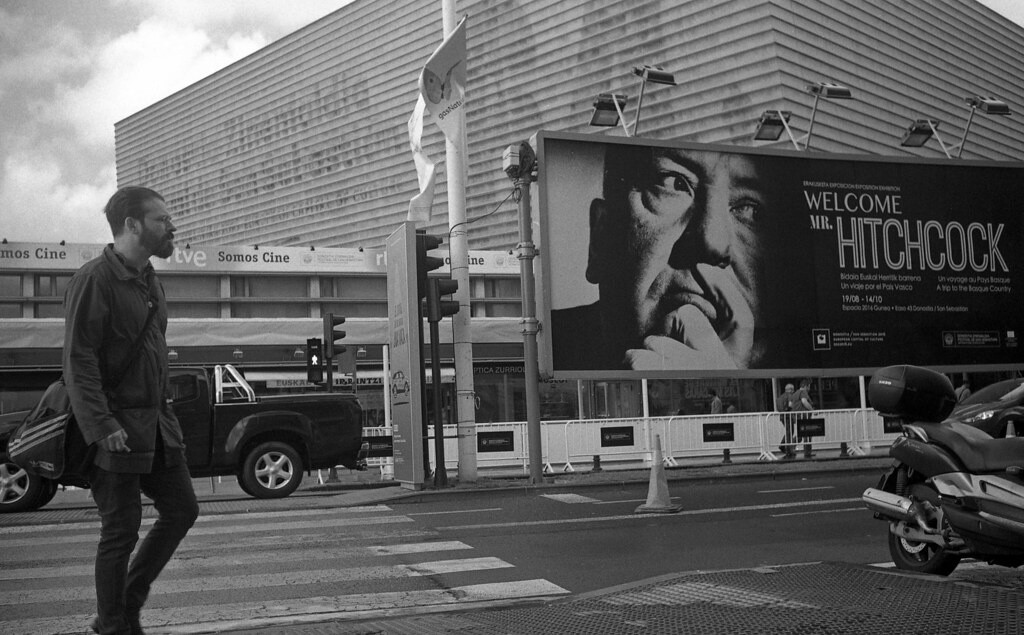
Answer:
<box><xmin>726</xmin><ymin>400</ymin><xmax>738</xmax><ymax>413</ymax></box>
<box><xmin>708</xmin><ymin>390</ymin><xmax>722</xmax><ymax>414</ymax></box>
<box><xmin>61</xmin><ymin>186</ymin><xmax>199</xmax><ymax>635</ymax></box>
<box><xmin>776</xmin><ymin>378</ymin><xmax>817</xmax><ymax>457</ymax></box>
<box><xmin>550</xmin><ymin>142</ymin><xmax>775</xmax><ymax>370</ymax></box>
<box><xmin>955</xmin><ymin>380</ymin><xmax>971</xmax><ymax>401</ymax></box>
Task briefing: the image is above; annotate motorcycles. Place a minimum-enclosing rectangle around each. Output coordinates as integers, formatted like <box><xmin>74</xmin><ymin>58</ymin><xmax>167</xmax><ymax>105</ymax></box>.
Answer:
<box><xmin>862</xmin><ymin>364</ymin><xmax>1024</xmax><ymax>578</ymax></box>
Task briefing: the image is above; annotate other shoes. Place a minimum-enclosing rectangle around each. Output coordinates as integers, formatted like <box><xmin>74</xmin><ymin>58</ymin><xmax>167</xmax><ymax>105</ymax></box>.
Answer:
<box><xmin>804</xmin><ymin>453</ymin><xmax>816</xmax><ymax>457</ymax></box>
<box><xmin>90</xmin><ymin>610</ymin><xmax>146</xmax><ymax>635</ymax></box>
<box><xmin>779</xmin><ymin>446</ymin><xmax>787</xmax><ymax>455</ymax></box>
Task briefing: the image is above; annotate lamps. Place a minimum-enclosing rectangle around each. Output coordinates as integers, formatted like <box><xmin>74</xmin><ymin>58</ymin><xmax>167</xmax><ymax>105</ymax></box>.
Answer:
<box><xmin>899</xmin><ymin>116</ymin><xmax>952</xmax><ymax>158</ymax></box>
<box><xmin>588</xmin><ymin>92</ymin><xmax>631</xmax><ymax>137</ymax></box>
<box><xmin>629</xmin><ymin>62</ymin><xmax>677</xmax><ymax>135</ymax></box>
<box><xmin>2</xmin><ymin>237</ymin><xmax>8</xmax><ymax>244</ymax></box>
<box><xmin>59</xmin><ymin>240</ymin><xmax>65</xmax><ymax>245</ymax></box>
<box><xmin>310</xmin><ymin>245</ymin><xmax>316</xmax><ymax>251</ymax></box>
<box><xmin>185</xmin><ymin>242</ymin><xmax>191</xmax><ymax>249</ymax></box>
<box><xmin>248</xmin><ymin>244</ymin><xmax>258</xmax><ymax>250</ymax></box>
<box><xmin>293</xmin><ymin>346</ymin><xmax>304</xmax><ymax>358</ymax></box>
<box><xmin>231</xmin><ymin>346</ymin><xmax>244</xmax><ymax>359</ymax></box>
<box><xmin>802</xmin><ymin>78</ymin><xmax>852</xmax><ymax>149</ymax></box>
<box><xmin>956</xmin><ymin>91</ymin><xmax>1013</xmax><ymax>159</ymax></box>
<box><xmin>356</xmin><ymin>346</ymin><xmax>368</xmax><ymax>358</ymax></box>
<box><xmin>168</xmin><ymin>349</ymin><xmax>179</xmax><ymax>360</ymax></box>
<box><xmin>358</xmin><ymin>246</ymin><xmax>364</xmax><ymax>252</ymax></box>
<box><xmin>752</xmin><ymin>108</ymin><xmax>801</xmax><ymax>150</ymax></box>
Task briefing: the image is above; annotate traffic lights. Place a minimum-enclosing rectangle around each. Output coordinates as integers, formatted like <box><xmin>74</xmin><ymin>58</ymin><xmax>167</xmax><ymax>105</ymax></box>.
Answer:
<box><xmin>323</xmin><ymin>312</ymin><xmax>347</xmax><ymax>357</ymax></box>
<box><xmin>416</xmin><ymin>229</ymin><xmax>444</xmax><ymax>297</ymax></box>
<box><xmin>425</xmin><ymin>278</ymin><xmax>459</xmax><ymax>323</ymax></box>
<box><xmin>307</xmin><ymin>338</ymin><xmax>323</xmax><ymax>382</ymax></box>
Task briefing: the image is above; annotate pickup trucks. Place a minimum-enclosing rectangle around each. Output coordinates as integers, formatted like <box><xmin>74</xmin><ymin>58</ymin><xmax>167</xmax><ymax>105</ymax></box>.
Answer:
<box><xmin>0</xmin><ymin>363</ymin><xmax>362</xmax><ymax>513</ymax></box>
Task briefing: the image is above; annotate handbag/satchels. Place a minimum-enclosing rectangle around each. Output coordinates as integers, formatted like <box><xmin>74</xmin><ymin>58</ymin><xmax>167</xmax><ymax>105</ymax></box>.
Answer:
<box><xmin>6</xmin><ymin>376</ymin><xmax>101</xmax><ymax>487</ymax></box>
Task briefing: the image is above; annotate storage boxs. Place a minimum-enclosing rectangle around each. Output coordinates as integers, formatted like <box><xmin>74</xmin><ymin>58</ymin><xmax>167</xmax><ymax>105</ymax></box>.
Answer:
<box><xmin>868</xmin><ymin>358</ymin><xmax>963</xmax><ymax>422</ymax></box>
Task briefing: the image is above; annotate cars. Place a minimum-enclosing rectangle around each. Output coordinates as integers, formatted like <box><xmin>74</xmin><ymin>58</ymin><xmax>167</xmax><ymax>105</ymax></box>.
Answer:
<box><xmin>945</xmin><ymin>375</ymin><xmax>1024</xmax><ymax>439</ymax></box>
